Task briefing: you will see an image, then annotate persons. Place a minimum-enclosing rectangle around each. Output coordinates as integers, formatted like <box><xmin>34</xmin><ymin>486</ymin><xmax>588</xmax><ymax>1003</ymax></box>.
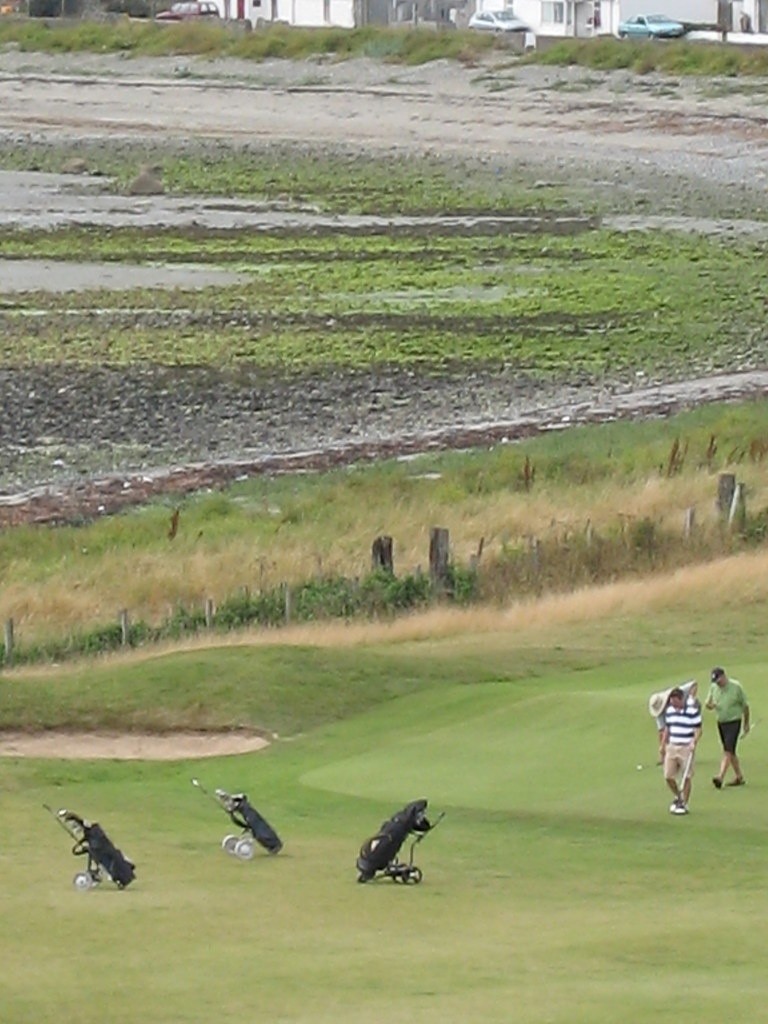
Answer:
<box><xmin>705</xmin><ymin>667</ymin><xmax>750</xmax><ymax>789</ymax></box>
<box><xmin>648</xmin><ymin>679</ymin><xmax>702</xmax><ymax>814</ymax></box>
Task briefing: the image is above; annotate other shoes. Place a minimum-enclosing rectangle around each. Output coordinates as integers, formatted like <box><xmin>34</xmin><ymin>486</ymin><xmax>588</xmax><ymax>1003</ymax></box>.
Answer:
<box><xmin>670</xmin><ymin>799</ymin><xmax>677</xmax><ymax>813</ymax></box>
<box><xmin>726</xmin><ymin>778</ymin><xmax>746</xmax><ymax>786</ymax></box>
<box><xmin>712</xmin><ymin>776</ymin><xmax>722</xmax><ymax>789</ymax></box>
<box><xmin>674</xmin><ymin>801</ymin><xmax>686</xmax><ymax>815</ymax></box>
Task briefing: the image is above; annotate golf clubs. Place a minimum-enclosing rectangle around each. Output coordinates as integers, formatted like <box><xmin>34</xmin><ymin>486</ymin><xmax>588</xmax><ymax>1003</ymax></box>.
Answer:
<box><xmin>636</xmin><ymin>764</ymin><xmax>657</xmax><ymax>770</ymax></box>
<box><xmin>190</xmin><ymin>776</ymin><xmax>229</xmax><ymax>814</ymax></box>
<box><xmin>741</xmin><ymin>717</ymin><xmax>762</xmax><ymax>740</ymax></box>
<box><xmin>41</xmin><ymin>803</ymin><xmax>85</xmax><ymax>848</ymax></box>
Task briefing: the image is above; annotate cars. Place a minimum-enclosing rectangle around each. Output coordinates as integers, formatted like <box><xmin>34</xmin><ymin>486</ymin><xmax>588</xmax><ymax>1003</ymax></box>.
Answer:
<box><xmin>615</xmin><ymin>14</ymin><xmax>685</xmax><ymax>40</ymax></box>
<box><xmin>155</xmin><ymin>0</ymin><xmax>220</xmax><ymax>22</ymax></box>
<box><xmin>467</xmin><ymin>9</ymin><xmax>529</xmax><ymax>33</ymax></box>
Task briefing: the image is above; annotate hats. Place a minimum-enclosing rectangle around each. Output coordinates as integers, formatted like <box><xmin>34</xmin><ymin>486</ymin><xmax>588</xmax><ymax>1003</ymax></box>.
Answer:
<box><xmin>648</xmin><ymin>692</ymin><xmax>667</xmax><ymax>717</ymax></box>
<box><xmin>710</xmin><ymin>667</ymin><xmax>724</xmax><ymax>683</ymax></box>
